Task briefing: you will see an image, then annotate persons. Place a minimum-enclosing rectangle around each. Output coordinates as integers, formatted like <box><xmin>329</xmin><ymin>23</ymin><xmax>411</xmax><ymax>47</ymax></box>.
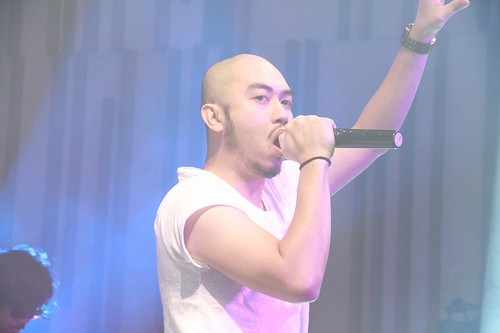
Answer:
<box><xmin>154</xmin><ymin>1</ymin><xmax>469</xmax><ymax>333</ymax></box>
<box><xmin>0</xmin><ymin>249</ymin><xmax>53</xmax><ymax>333</ymax></box>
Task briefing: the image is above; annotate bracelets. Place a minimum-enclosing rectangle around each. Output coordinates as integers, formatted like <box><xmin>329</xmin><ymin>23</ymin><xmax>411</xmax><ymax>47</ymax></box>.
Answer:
<box><xmin>299</xmin><ymin>156</ymin><xmax>331</xmax><ymax>170</ymax></box>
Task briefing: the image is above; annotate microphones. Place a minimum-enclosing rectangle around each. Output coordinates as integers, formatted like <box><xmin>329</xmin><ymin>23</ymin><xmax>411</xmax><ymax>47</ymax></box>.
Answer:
<box><xmin>333</xmin><ymin>129</ymin><xmax>404</xmax><ymax>148</ymax></box>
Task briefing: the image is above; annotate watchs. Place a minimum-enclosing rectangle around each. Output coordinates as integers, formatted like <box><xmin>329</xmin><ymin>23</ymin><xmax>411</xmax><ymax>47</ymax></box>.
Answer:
<box><xmin>400</xmin><ymin>23</ymin><xmax>436</xmax><ymax>53</ymax></box>
